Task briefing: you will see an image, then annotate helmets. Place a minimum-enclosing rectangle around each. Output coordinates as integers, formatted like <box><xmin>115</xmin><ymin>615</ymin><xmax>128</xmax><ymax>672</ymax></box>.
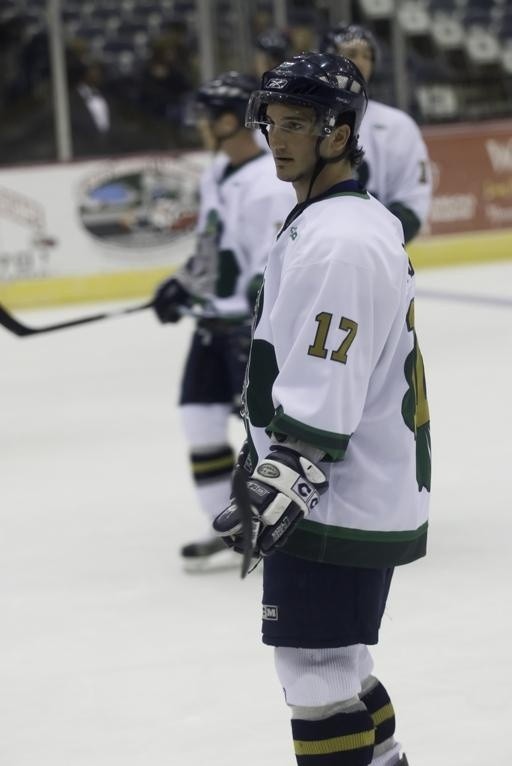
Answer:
<box><xmin>255</xmin><ymin>49</ymin><xmax>371</xmax><ymax>157</ymax></box>
<box><xmin>195</xmin><ymin>68</ymin><xmax>265</xmax><ymax>133</ymax></box>
<box><xmin>320</xmin><ymin>20</ymin><xmax>381</xmax><ymax>76</ymax></box>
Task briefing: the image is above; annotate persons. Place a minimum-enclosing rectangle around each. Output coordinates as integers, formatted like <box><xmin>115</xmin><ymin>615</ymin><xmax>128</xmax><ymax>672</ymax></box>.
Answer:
<box><xmin>330</xmin><ymin>21</ymin><xmax>435</xmax><ymax>248</ymax></box>
<box><xmin>213</xmin><ymin>47</ymin><xmax>435</xmax><ymax>766</ymax></box>
<box><xmin>148</xmin><ymin>67</ymin><xmax>299</xmax><ymax>564</ymax></box>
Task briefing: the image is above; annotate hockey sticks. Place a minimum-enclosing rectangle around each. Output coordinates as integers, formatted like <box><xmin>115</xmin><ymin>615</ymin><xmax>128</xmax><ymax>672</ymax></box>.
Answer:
<box><xmin>232</xmin><ymin>408</ymin><xmax>261</xmax><ymax>577</ymax></box>
<box><xmin>0</xmin><ymin>295</ymin><xmax>156</xmax><ymax>335</ymax></box>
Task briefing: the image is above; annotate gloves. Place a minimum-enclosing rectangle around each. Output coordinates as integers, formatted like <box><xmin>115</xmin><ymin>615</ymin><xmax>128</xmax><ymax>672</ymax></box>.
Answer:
<box><xmin>153</xmin><ymin>277</ymin><xmax>196</xmax><ymax>325</ymax></box>
<box><xmin>211</xmin><ymin>445</ymin><xmax>330</xmax><ymax>562</ymax></box>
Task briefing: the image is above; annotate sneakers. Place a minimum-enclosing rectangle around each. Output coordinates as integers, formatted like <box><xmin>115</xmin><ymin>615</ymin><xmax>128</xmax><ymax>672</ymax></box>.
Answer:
<box><xmin>182</xmin><ymin>524</ymin><xmax>232</xmax><ymax>559</ymax></box>
